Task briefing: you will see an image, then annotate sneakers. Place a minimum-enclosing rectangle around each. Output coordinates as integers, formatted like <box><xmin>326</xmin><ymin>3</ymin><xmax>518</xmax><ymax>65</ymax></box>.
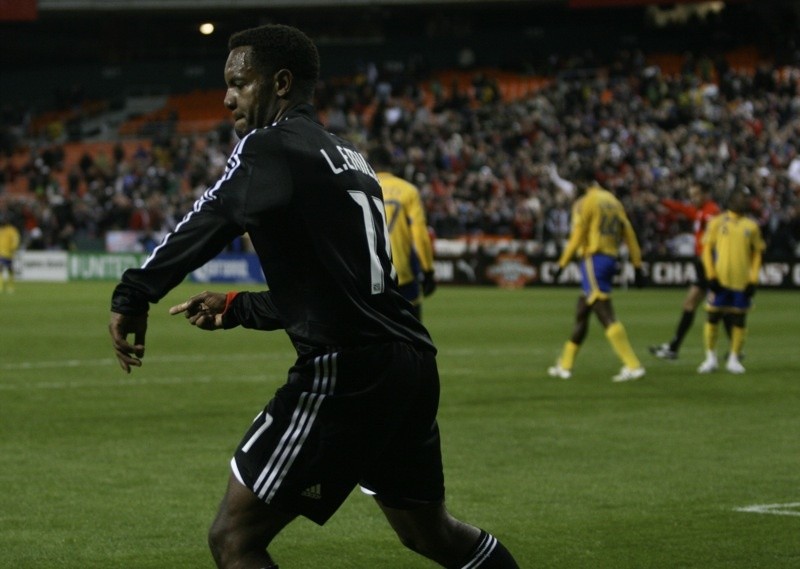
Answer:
<box><xmin>648</xmin><ymin>344</ymin><xmax>746</xmax><ymax>374</ymax></box>
<box><xmin>610</xmin><ymin>366</ymin><xmax>646</xmax><ymax>382</ymax></box>
<box><xmin>548</xmin><ymin>364</ymin><xmax>572</xmax><ymax>379</ymax></box>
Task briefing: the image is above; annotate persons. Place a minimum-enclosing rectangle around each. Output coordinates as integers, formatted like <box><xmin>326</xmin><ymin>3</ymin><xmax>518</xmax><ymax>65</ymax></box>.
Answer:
<box><xmin>0</xmin><ymin>43</ymin><xmax>800</xmax><ymax>382</ymax></box>
<box><xmin>110</xmin><ymin>25</ymin><xmax>521</xmax><ymax>569</ymax></box>
